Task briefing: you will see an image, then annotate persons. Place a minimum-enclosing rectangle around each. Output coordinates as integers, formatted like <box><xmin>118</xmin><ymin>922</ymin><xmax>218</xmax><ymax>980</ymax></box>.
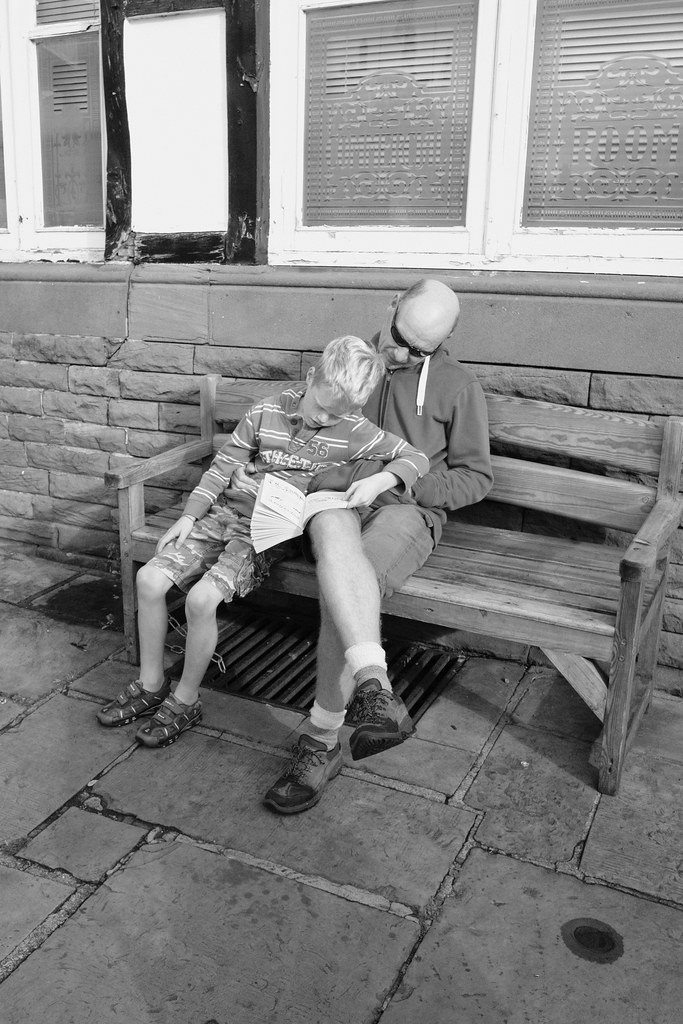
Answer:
<box><xmin>96</xmin><ymin>336</ymin><xmax>430</xmax><ymax>748</ymax></box>
<box><xmin>262</xmin><ymin>279</ymin><xmax>494</xmax><ymax>814</ymax></box>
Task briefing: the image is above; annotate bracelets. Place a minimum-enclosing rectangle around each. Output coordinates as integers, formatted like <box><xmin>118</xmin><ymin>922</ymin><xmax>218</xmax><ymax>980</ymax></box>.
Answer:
<box><xmin>184</xmin><ymin>514</ymin><xmax>194</xmax><ymax>522</ymax></box>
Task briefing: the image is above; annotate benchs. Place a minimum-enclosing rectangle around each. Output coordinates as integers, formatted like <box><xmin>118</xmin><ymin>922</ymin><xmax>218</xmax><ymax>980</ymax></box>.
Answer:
<box><xmin>111</xmin><ymin>371</ymin><xmax>682</xmax><ymax>797</ymax></box>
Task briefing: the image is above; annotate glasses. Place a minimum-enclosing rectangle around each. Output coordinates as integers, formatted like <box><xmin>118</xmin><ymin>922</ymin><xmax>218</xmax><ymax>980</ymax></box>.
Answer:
<box><xmin>390</xmin><ymin>306</ymin><xmax>442</xmax><ymax>357</ymax></box>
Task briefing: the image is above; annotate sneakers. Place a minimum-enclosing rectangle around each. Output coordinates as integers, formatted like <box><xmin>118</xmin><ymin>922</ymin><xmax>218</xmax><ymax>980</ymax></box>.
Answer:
<box><xmin>262</xmin><ymin>733</ymin><xmax>341</xmax><ymax>813</ymax></box>
<box><xmin>345</xmin><ymin>678</ymin><xmax>417</xmax><ymax>761</ymax></box>
<box><xmin>135</xmin><ymin>692</ymin><xmax>203</xmax><ymax>748</ymax></box>
<box><xmin>95</xmin><ymin>677</ymin><xmax>171</xmax><ymax>728</ymax></box>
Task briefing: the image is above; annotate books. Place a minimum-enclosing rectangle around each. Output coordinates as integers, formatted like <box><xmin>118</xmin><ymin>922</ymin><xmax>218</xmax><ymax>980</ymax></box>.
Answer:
<box><xmin>250</xmin><ymin>473</ymin><xmax>349</xmax><ymax>554</ymax></box>
